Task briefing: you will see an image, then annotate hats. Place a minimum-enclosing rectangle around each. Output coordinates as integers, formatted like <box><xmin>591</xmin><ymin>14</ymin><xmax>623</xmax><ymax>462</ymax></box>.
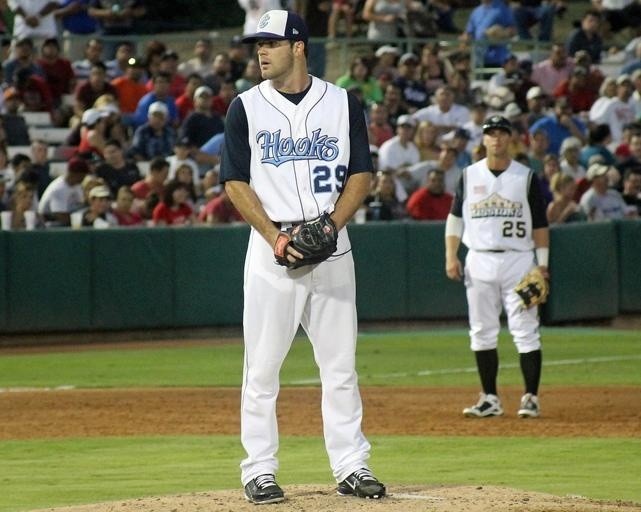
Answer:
<box><xmin>375</xmin><ymin>44</ymin><xmax>631</xmax><ymax>182</ymax></box>
<box><xmin>241</xmin><ymin>10</ymin><xmax>309</xmax><ymax>42</ymax></box>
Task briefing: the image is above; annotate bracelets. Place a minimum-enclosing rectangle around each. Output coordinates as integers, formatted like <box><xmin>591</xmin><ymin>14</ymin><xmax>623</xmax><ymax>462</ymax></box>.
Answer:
<box><xmin>535</xmin><ymin>246</ymin><xmax>551</xmax><ymax>268</ymax></box>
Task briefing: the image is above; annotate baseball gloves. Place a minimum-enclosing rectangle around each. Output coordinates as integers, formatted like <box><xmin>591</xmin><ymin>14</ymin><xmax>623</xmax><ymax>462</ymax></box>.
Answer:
<box><xmin>516</xmin><ymin>269</ymin><xmax>549</xmax><ymax>309</ymax></box>
<box><xmin>290</xmin><ymin>213</ymin><xmax>338</xmax><ymax>269</ymax></box>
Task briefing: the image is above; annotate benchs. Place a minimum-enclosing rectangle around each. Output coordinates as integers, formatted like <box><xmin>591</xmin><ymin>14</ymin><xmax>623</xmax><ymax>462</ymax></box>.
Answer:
<box><xmin>0</xmin><ymin>93</ymin><xmax>154</xmax><ymax>180</ymax></box>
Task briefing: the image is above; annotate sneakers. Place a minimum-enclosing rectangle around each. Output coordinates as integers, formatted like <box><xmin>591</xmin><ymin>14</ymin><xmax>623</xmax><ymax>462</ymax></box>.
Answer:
<box><xmin>463</xmin><ymin>392</ymin><xmax>503</xmax><ymax>416</ymax></box>
<box><xmin>336</xmin><ymin>468</ymin><xmax>386</xmax><ymax>497</ymax></box>
<box><xmin>245</xmin><ymin>473</ymin><xmax>284</xmax><ymax>505</ymax></box>
<box><xmin>69</xmin><ymin>86</ymin><xmax>213</xmax><ymax>202</ymax></box>
<box><xmin>517</xmin><ymin>393</ymin><xmax>540</xmax><ymax>416</ymax></box>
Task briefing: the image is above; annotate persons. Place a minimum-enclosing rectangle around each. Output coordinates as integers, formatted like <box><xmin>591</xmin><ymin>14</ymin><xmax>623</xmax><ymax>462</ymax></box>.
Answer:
<box><xmin>1</xmin><ymin>1</ymin><xmax>640</xmax><ymax>232</ymax></box>
<box><xmin>443</xmin><ymin>114</ymin><xmax>552</xmax><ymax>421</ymax></box>
<box><xmin>221</xmin><ymin>10</ymin><xmax>387</xmax><ymax>503</ymax></box>
